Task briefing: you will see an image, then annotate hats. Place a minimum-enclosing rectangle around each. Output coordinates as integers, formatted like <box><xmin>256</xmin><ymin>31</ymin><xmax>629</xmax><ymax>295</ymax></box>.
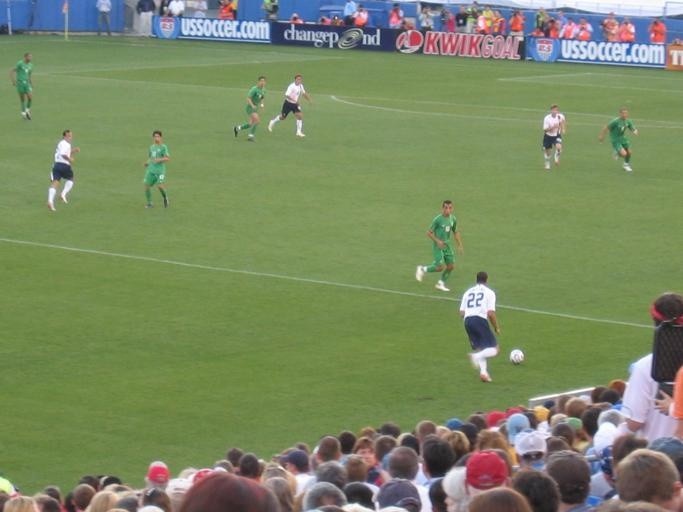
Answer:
<box><xmin>280</xmin><ymin>450</ymin><xmax>308</xmax><ymax>464</ymax></box>
<box><xmin>467</xmin><ymin>451</ymin><xmax>507</xmax><ymax>489</ymax></box>
<box><xmin>489</xmin><ymin>405</ymin><xmax>583</xmax><ymax>454</ymax></box>
<box><xmin>372</xmin><ymin>479</ymin><xmax>421</xmax><ymax>512</ymax></box>
<box><xmin>149</xmin><ymin>461</ymin><xmax>168</xmax><ymax>483</ymax></box>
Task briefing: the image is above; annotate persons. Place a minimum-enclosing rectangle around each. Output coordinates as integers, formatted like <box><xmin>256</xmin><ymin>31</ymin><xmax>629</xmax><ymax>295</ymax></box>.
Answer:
<box><xmin>96</xmin><ymin>1</ymin><xmax>112</xmax><ymax>36</ymax></box>
<box><xmin>6</xmin><ymin>53</ymin><xmax>33</xmax><ymax>120</ymax></box>
<box><xmin>194</xmin><ymin>2</ymin><xmax>208</xmax><ymax>18</ymax></box>
<box><xmin>291</xmin><ymin>13</ymin><xmax>304</xmax><ymax>24</ymax></box>
<box><xmin>222</xmin><ymin>0</ymin><xmax>237</xmax><ymax>19</ymax></box>
<box><xmin>441</xmin><ymin>2</ymin><xmax>506</xmax><ymax>36</ymax></box>
<box><xmin>419</xmin><ymin>4</ymin><xmax>434</xmax><ymax>31</ymax></box>
<box><xmin>141</xmin><ymin>131</ymin><xmax>168</xmax><ymax>207</ymax></box>
<box><xmin>414</xmin><ymin>197</ymin><xmax>465</xmax><ymax>291</ymax></box>
<box><xmin>267</xmin><ymin>75</ymin><xmax>311</xmax><ymax>136</ymax></box>
<box><xmin>457</xmin><ymin>271</ymin><xmax>501</xmax><ymax>383</ymax></box>
<box><xmin>233</xmin><ymin>75</ymin><xmax>266</xmax><ymax>142</ymax></box>
<box><xmin>44</xmin><ymin>131</ymin><xmax>78</xmax><ymax>210</ymax></box>
<box><xmin>261</xmin><ymin>1</ymin><xmax>279</xmax><ymax>21</ymax></box>
<box><xmin>622</xmin><ymin>292</ymin><xmax>682</xmax><ymax>440</ymax></box>
<box><xmin>604</xmin><ymin>12</ymin><xmax>636</xmax><ymax>41</ymax></box>
<box><xmin>320</xmin><ymin>1</ymin><xmax>369</xmax><ymax>27</ymax></box>
<box><xmin>648</xmin><ymin>18</ymin><xmax>667</xmax><ymax>43</ymax></box>
<box><xmin>136</xmin><ymin>0</ymin><xmax>185</xmax><ymax>38</ymax></box>
<box><xmin>509</xmin><ymin>10</ymin><xmax>525</xmax><ymax>41</ymax></box>
<box><xmin>538</xmin><ymin>103</ymin><xmax>564</xmax><ymax>170</ymax></box>
<box><xmin>0</xmin><ymin>369</ymin><xmax>683</xmax><ymax>512</ymax></box>
<box><xmin>531</xmin><ymin>7</ymin><xmax>594</xmax><ymax>41</ymax></box>
<box><xmin>598</xmin><ymin>108</ymin><xmax>639</xmax><ymax>171</ymax></box>
<box><xmin>388</xmin><ymin>3</ymin><xmax>404</xmax><ymax>30</ymax></box>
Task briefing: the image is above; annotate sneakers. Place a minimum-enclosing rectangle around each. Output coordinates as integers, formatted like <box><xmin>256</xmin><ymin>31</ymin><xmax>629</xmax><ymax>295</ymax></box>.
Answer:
<box><xmin>416</xmin><ymin>265</ymin><xmax>423</xmax><ymax>282</ymax></box>
<box><xmin>296</xmin><ymin>131</ymin><xmax>305</xmax><ymax>137</ymax></box>
<box><xmin>47</xmin><ymin>193</ymin><xmax>67</xmax><ymax>211</ymax></box>
<box><xmin>435</xmin><ymin>282</ymin><xmax>450</xmax><ymax>291</ymax></box>
<box><xmin>233</xmin><ymin>126</ymin><xmax>254</xmax><ymax>142</ymax></box>
<box><xmin>268</xmin><ymin>120</ymin><xmax>273</xmax><ymax>131</ymax></box>
<box><xmin>469</xmin><ymin>353</ymin><xmax>492</xmax><ymax>382</ymax></box>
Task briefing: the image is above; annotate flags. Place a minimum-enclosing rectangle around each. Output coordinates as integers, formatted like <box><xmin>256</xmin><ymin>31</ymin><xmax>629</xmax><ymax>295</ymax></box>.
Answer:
<box><xmin>61</xmin><ymin>2</ymin><xmax>69</xmax><ymax>14</ymax></box>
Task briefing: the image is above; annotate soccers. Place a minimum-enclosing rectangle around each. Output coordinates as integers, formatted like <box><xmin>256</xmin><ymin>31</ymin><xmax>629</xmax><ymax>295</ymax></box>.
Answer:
<box><xmin>512</xmin><ymin>349</ymin><xmax>526</xmax><ymax>368</ymax></box>
